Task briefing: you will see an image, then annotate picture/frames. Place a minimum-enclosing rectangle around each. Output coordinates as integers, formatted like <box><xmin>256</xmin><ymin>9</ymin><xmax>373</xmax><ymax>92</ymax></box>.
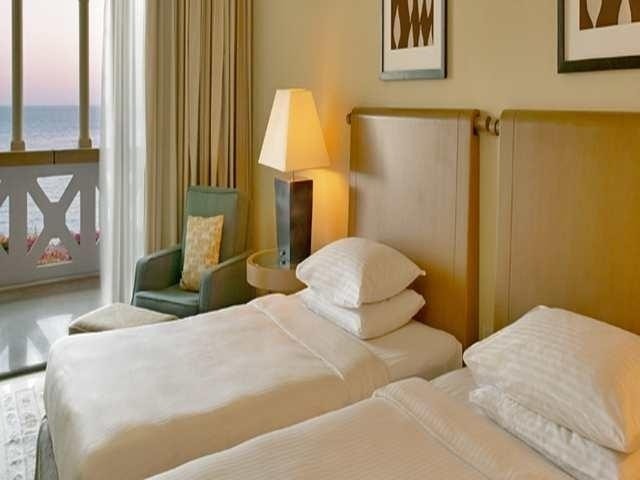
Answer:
<box><xmin>379</xmin><ymin>0</ymin><xmax>447</xmax><ymax>81</ymax></box>
<box><xmin>556</xmin><ymin>0</ymin><xmax>640</xmax><ymax>73</ymax></box>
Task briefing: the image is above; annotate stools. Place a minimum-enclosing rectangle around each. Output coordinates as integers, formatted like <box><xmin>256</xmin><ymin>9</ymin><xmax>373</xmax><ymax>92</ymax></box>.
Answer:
<box><xmin>69</xmin><ymin>303</ymin><xmax>179</xmax><ymax>336</ymax></box>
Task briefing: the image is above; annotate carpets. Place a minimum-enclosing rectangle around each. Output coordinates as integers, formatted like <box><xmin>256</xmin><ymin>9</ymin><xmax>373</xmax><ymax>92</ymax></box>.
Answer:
<box><xmin>0</xmin><ymin>359</ymin><xmax>47</xmax><ymax>480</ymax></box>
<box><xmin>145</xmin><ymin>110</ymin><xmax>640</xmax><ymax>480</ymax></box>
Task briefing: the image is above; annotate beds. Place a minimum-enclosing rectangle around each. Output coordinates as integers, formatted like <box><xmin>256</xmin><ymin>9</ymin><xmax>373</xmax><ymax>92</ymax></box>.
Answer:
<box><xmin>35</xmin><ymin>107</ymin><xmax>491</xmax><ymax>480</ymax></box>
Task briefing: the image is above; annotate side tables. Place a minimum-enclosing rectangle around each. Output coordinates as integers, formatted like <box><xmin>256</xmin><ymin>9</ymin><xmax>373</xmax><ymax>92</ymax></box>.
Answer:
<box><xmin>247</xmin><ymin>249</ymin><xmax>307</xmax><ymax>294</ymax></box>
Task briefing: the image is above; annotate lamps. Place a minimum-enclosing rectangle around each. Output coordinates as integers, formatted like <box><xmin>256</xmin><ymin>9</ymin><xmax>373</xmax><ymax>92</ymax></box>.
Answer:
<box><xmin>257</xmin><ymin>88</ymin><xmax>330</xmax><ymax>268</ymax></box>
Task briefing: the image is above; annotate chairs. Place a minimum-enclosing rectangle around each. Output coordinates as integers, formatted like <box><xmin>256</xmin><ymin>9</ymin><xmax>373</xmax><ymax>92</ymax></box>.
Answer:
<box><xmin>130</xmin><ymin>186</ymin><xmax>256</xmax><ymax>319</ymax></box>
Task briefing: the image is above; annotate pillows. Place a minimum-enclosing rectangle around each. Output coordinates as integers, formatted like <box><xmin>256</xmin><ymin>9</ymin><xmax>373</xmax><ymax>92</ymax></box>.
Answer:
<box><xmin>462</xmin><ymin>304</ymin><xmax>640</xmax><ymax>454</ymax></box>
<box><xmin>295</xmin><ymin>289</ymin><xmax>426</xmax><ymax>340</ymax></box>
<box><xmin>469</xmin><ymin>388</ymin><xmax>640</xmax><ymax>480</ymax></box>
<box><xmin>180</xmin><ymin>215</ymin><xmax>224</xmax><ymax>291</ymax></box>
<box><xmin>295</xmin><ymin>238</ymin><xmax>426</xmax><ymax>309</ymax></box>
